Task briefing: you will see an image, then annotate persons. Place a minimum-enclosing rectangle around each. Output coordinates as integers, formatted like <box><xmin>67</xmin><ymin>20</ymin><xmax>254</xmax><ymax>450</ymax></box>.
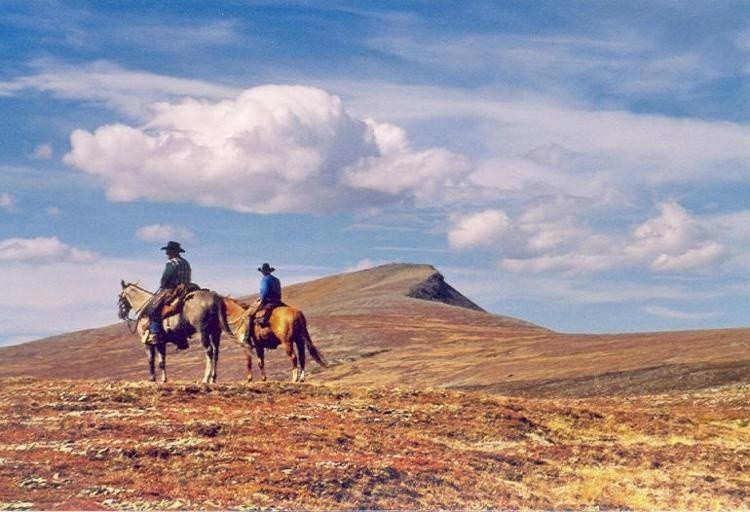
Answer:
<box><xmin>146</xmin><ymin>240</ymin><xmax>191</xmax><ymax>344</ymax></box>
<box><xmin>255</xmin><ymin>263</ymin><xmax>283</xmax><ymax>309</ymax></box>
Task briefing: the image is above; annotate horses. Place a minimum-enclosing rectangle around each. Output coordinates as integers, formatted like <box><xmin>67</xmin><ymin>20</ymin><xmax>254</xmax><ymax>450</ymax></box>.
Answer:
<box><xmin>221</xmin><ymin>292</ymin><xmax>329</xmax><ymax>382</ymax></box>
<box><xmin>117</xmin><ymin>279</ymin><xmax>234</xmax><ymax>384</ymax></box>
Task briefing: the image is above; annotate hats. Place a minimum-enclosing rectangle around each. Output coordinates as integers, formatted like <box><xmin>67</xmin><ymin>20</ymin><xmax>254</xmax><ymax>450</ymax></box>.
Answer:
<box><xmin>161</xmin><ymin>241</ymin><xmax>185</xmax><ymax>253</ymax></box>
<box><xmin>258</xmin><ymin>263</ymin><xmax>275</xmax><ymax>273</ymax></box>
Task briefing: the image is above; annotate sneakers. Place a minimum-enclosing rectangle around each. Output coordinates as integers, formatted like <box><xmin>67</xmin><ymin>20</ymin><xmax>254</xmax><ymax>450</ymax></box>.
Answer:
<box><xmin>146</xmin><ymin>334</ymin><xmax>158</xmax><ymax>345</ymax></box>
